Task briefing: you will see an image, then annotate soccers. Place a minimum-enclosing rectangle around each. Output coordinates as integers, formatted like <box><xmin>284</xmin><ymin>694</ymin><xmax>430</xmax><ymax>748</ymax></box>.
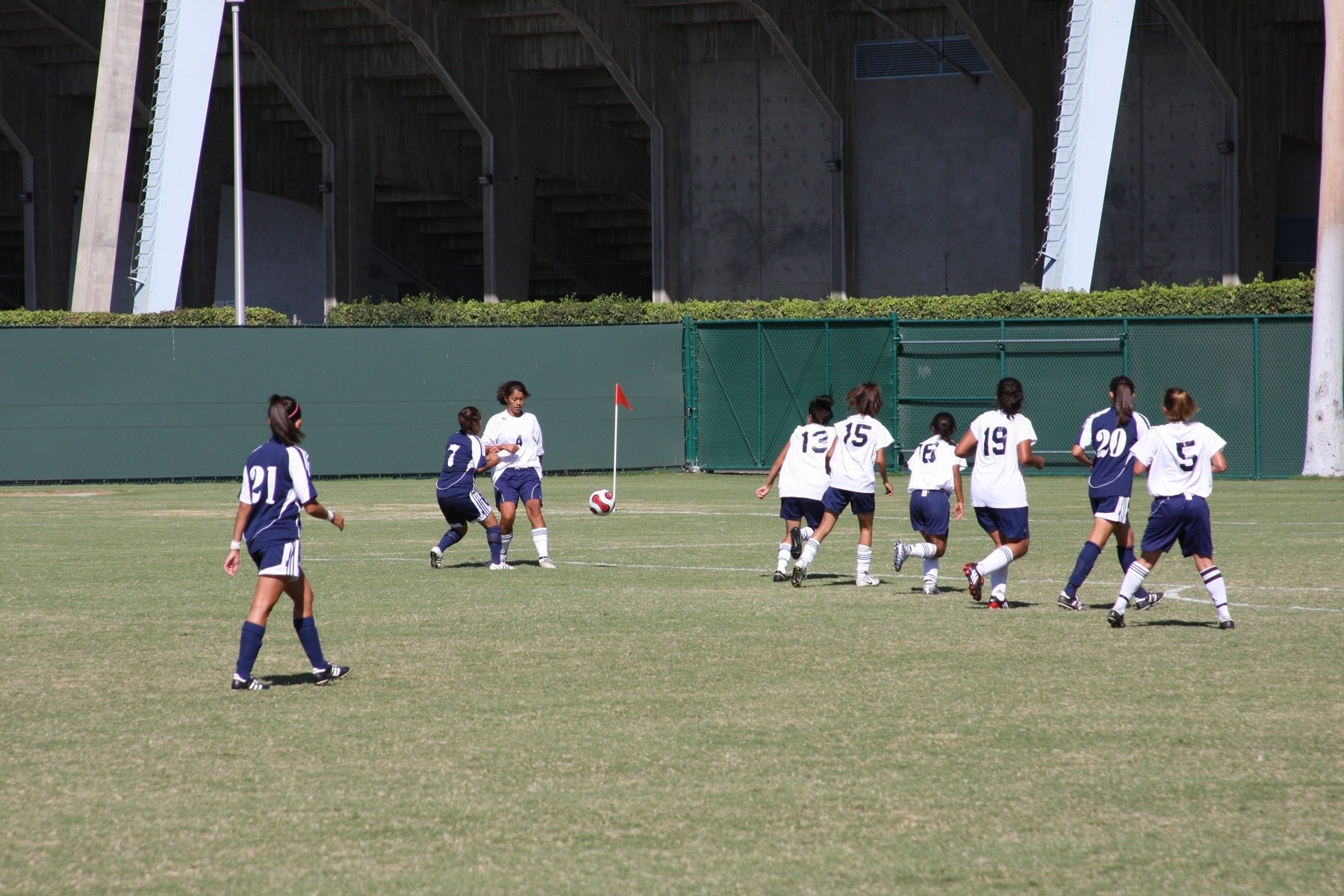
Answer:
<box><xmin>589</xmin><ymin>490</ymin><xmax>615</xmax><ymax>516</ymax></box>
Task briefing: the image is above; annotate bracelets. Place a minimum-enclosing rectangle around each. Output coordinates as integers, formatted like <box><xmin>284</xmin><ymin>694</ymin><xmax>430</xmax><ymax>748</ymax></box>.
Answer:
<box><xmin>326</xmin><ymin>510</ymin><xmax>334</xmax><ymax>520</ymax></box>
<box><xmin>231</xmin><ymin>541</ymin><xmax>241</xmax><ymax>550</ymax></box>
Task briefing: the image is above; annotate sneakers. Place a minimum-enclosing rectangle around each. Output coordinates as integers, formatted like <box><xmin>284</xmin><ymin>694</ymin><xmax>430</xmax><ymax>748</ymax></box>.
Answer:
<box><xmin>311</xmin><ymin>662</ymin><xmax>350</xmax><ymax>686</ymax></box>
<box><xmin>964</xmin><ymin>562</ymin><xmax>983</xmax><ymax>601</ymax></box>
<box><xmin>538</xmin><ymin>558</ymin><xmax>557</xmax><ymax>570</ymax></box>
<box><xmin>894</xmin><ymin>540</ymin><xmax>910</xmax><ymax>572</ymax></box>
<box><xmin>232</xmin><ymin>674</ymin><xmax>269</xmax><ymax>690</ymax></box>
<box><xmin>1135</xmin><ymin>591</ymin><xmax>1165</xmax><ymax>610</ymax></box>
<box><xmin>500</xmin><ymin>553</ymin><xmax>507</xmax><ymax>562</ymax></box>
<box><xmin>1056</xmin><ymin>591</ymin><xmax>1085</xmax><ymax>611</ymax></box>
<box><xmin>1107</xmin><ymin>610</ymin><xmax>1126</xmax><ymax>628</ymax></box>
<box><xmin>790</xmin><ymin>526</ymin><xmax>801</xmax><ymax>559</ymax></box>
<box><xmin>430</xmin><ymin>546</ymin><xmax>443</xmax><ymax>568</ymax></box>
<box><xmin>922</xmin><ymin>584</ymin><xmax>943</xmax><ymax>595</ymax></box>
<box><xmin>792</xmin><ymin>561</ymin><xmax>807</xmax><ymax>588</ymax></box>
<box><xmin>1219</xmin><ymin>618</ymin><xmax>1234</xmax><ymax>631</ymax></box>
<box><xmin>988</xmin><ymin>597</ymin><xmax>1015</xmax><ymax>609</ymax></box>
<box><xmin>773</xmin><ymin>571</ymin><xmax>787</xmax><ymax>581</ymax></box>
<box><xmin>856</xmin><ymin>574</ymin><xmax>880</xmax><ymax>588</ymax></box>
<box><xmin>489</xmin><ymin>561</ymin><xmax>516</xmax><ymax>570</ymax></box>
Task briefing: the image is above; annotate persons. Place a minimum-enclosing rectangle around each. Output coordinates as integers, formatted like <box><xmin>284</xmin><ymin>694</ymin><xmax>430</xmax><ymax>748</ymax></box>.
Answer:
<box><xmin>791</xmin><ymin>381</ymin><xmax>895</xmax><ymax>589</ymax></box>
<box><xmin>481</xmin><ymin>381</ymin><xmax>557</xmax><ymax>569</ymax></box>
<box><xmin>954</xmin><ymin>377</ymin><xmax>1043</xmax><ymax>609</ymax></box>
<box><xmin>1107</xmin><ymin>386</ymin><xmax>1234</xmax><ymax>631</ymax></box>
<box><xmin>755</xmin><ymin>394</ymin><xmax>835</xmax><ymax>581</ymax></box>
<box><xmin>891</xmin><ymin>412</ymin><xmax>968</xmax><ymax>594</ymax></box>
<box><xmin>1057</xmin><ymin>373</ymin><xmax>1165</xmax><ymax>612</ymax></box>
<box><xmin>224</xmin><ymin>393</ymin><xmax>351</xmax><ymax>691</ymax></box>
<box><xmin>430</xmin><ymin>406</ymin><xmax>516</xmax><ymax>570</ymax></box>
